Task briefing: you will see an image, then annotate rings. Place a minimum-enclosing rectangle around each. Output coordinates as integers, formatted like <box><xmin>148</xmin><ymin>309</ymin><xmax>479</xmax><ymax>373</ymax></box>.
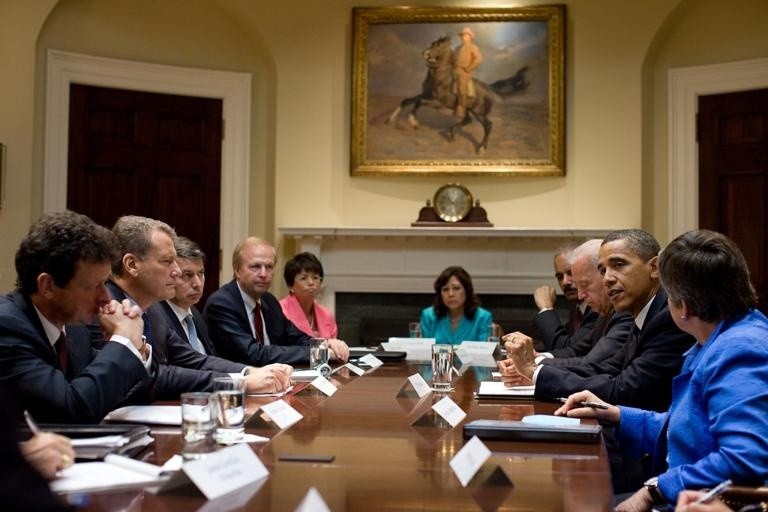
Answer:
<box><xmin>509</xmin><ymin>336</ymin><xmax>515</xmax><ymax>343</ymax></box>
<box><xmin>59</xmin><ymin>454</ymin><xmax>70</xmax><ymax>470</ymax></box>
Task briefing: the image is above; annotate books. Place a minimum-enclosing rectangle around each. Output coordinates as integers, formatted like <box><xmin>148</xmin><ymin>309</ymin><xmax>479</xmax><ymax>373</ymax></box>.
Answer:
<box><xmin>290</xmin><ymin>362</ymin><xmax>332</xmax><ymax>385</ymax></box>
<box><xmin>462</xmin><ymin>418</ymin><xmax>604</xmax><ymax>445</ymax></box>
<box><xmin>347</xmin><ymin>350</ymin><xmax>408</xmax><ymax>364</ymax></box>
<box><xmin>474</xmin><ymin>380</ymin><xmax>537</xmax><ymax>402</ymax></box>
<box><xmin>13</xmin><ymin>424</ymin><xmax>157</xmax><ymax>462</ymax></box>
<box><xmin>102</xmin><ymin>405</ymin><xmax>246</xmax><ymax>427</ymax></box>
<box><xmin>45</xmin><ymin>454</ymin><xmax>175</xmax><ymax>496</ymax></box>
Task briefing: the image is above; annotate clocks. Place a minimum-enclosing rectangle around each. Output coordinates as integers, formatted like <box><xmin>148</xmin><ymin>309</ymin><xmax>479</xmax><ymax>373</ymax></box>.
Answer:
<box><xmin>433</xmin><ymin>183</ymin><xmax>473</xmax><ymax>222</ymax></box>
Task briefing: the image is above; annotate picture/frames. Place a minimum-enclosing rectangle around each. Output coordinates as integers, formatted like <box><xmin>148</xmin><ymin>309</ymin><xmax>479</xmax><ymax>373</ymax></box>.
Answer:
<box><xmin>350</xmin><ymin>3</ymin><xmax>566</xmax><ymax>177</ymax></box>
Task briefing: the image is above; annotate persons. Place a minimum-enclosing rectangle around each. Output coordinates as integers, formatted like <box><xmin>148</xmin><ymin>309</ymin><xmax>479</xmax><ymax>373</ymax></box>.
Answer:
<box><xmin>451</xmin><ymin>27</ymin><xmax>482</xmax><ymax>118</ymax></box>
<box><xmin>506</xmin><ymin>238</ymin><xmax>634</xmax><ymax>368</ymax></box>
<box><xmin>532</xmin><ymin>240</ymin><xmax>599</xmax><ymax>352</ymax></box>
<box><xmin>416</xmin><ymin>265</ymin><xmax>493</xmax><ymax>345</ymax></box>
<box><xmin>278</xmin><ymin>250</ymin><xmax>339</xmax><ymax>342</ymax></box>
<box><xmin>495</xmin><ymin>229</ymin><xmax>698</xmax><ymax>494</ymax></box>
<box><xmin>204</xmin><ymin>236</ymin><xmax>350</xmax><ymax>366</ymax></box>
<box><xmin>147</xmin><ymin>235</ymin><xmax>217</xmax><ymax>357</ymax></box>
<box><xmin>553</xmin><ymin>229</ymin><xmax>768</xmax><ymax>512</ymax></box>
<box><xmin>674</xmin><ymin>490</ymin><xmax>768</xmax><ymax>512</ymax></box>
<box><xmin>1</xmin><ymin>430</ymin><xmax>77</xmax><ymax>511</ymax></box>
<box><xmin>85</xmin><ymin>215</ymin><xmax>294</xmax><ymax>401</ymax></box>
<box><xmin>1</xmin><ymin>208</ymin><xmax>160</xmax><ymax>424</ymax></box>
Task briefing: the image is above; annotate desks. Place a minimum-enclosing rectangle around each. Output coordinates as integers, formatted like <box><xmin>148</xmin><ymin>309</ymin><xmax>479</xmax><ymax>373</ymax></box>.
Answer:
<box><xmin>49</xmin><ymin>358</ymin><xmax>613</xmax><ymax>512</ymax></box>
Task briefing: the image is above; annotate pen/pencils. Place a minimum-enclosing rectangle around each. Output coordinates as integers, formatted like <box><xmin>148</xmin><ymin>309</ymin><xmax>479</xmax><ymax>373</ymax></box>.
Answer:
<box><xmin>23</xmin><ymin>410</ymin><xmax>38</xmax><ymax>435</ymax></box>
<box><xmin>556</xmin><ymin>397</ymin><xmax>609</xmax><ymax>409</ymax></box>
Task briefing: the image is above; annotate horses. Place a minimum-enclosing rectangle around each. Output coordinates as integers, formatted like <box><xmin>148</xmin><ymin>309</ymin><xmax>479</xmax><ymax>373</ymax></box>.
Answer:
<box><xmin>387</xmin><ymin>34</ymin><xmax>537</xmax><ymax>155</ymax></box>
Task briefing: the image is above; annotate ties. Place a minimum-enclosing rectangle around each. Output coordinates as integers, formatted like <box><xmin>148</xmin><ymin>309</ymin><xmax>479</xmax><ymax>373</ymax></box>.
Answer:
<box><xmin>251</xmin><ymin>300</ymin><xmax>265</xmax><ymax>348</ymax></box>
<box><xmin>573</xmin><ymin>303</ymin><xmax>581</xmax><ymax>332</ymax></box>
<box><xmin>55</xmin><ymin>330</ymin><xmax>69</xmax><ymax>376</ymax></box>
<box><xmin>622</xmin><ymin>321</ymin><xmax>640</xmax><ymax>366</ymax></box>
<box><xmin>185</xmin><ymin>313</ymin><xmax>208</xmax><ymax>356</ymax></box>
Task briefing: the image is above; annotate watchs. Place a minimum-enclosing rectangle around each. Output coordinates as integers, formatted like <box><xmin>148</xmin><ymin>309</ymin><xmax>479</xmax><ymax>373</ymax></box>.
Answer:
<box><xmin>643</xmin><ymin>474</ymin><xmax>664</xmax><ymax>504</ymax></box>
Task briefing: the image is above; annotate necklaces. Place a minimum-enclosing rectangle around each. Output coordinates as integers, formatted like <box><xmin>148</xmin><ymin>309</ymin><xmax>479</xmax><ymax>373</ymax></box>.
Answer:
<box><xmin>449</xmin><ymin>317</ymin><xmax>459</xmax><ymax>324</ymax></box>
<box><xmin>310</xmin><ymin>309</ymin><xmax>314</xmax><ymax>330</ymax></box>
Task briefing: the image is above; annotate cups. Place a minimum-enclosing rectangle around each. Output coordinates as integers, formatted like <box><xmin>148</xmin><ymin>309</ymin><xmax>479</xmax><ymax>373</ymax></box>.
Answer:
<box><xmin>308</xmin><ymin>338</ymin><xmax>329</xmax><ymax>373</ymax></box>
<box><xmin>177</xmin><ymin>375</ymin><xmax>249</xmax><ymax>449</ymax></box>
<box><xmin>430</xmin><ymin>343</ymin><xmax>454</xmax><ymax>390</ymax></box>
<box><xmin>408</xmin><ymin>321</ymin><xmax>423</xmax><ymax>339</ymax></box>
<box><xmin>431</xmin><ymin>392</ymin><xmax>454</xmax><ymax>430</ymax></box>
